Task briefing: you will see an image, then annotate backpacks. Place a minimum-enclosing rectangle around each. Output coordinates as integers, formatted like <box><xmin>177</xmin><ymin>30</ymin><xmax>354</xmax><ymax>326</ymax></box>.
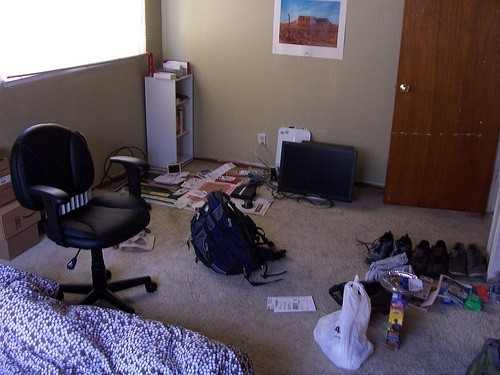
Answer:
<box><xmin>187</xmin><ymin>190</ymin><xmax>287</xmax><ymax>286</ymax></box>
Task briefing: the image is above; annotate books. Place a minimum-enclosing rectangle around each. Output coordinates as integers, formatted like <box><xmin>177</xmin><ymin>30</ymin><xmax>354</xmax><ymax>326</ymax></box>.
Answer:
<box><xmin>176</xmin><ymin>107</ymin><xmax>183</xmax><ymax>134</ymax></box>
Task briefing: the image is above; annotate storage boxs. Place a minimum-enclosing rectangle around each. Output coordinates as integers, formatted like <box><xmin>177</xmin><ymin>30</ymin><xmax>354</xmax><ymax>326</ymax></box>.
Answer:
<box><xmin>0</xmin><ymin>156</ymin><xmax>42</xmax><ymax>261</ymax></box>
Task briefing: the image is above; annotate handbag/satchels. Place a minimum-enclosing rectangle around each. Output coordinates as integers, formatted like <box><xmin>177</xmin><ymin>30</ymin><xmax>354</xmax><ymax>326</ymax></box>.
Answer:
<box><xmin>329</xmin><ymin>281</ymin><xmax>393</xmax><ymax>315</ymax></box>
<box><xmin>312</xmin><ymin>274</ymin><xmax>375</xmax><ymax>371</ymax></box>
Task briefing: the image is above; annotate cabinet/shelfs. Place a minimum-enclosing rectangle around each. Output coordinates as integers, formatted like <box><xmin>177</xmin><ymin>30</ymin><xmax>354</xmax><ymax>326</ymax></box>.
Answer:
<box><xmin>145</xmin><ymin>74</ymin><xmax>195</xmax><ymax>175</ymax></box>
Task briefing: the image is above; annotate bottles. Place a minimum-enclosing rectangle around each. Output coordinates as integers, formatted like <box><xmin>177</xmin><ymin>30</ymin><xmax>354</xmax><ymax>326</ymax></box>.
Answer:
<box><xmin>487</xmin><ymin>285</ymin><xmax>500</xmax><ymax>304</ymax></box>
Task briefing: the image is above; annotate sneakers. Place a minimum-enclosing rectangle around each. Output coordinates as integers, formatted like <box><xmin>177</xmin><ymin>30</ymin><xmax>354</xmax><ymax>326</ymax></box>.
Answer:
<box><xmin>365</xmin><ymin>230</ymin><xmax>487</xmax><ymax>278</ymax></box>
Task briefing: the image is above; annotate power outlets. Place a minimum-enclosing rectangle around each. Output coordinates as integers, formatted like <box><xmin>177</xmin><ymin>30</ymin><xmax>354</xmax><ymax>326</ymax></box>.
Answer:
<box><xmin>258</xmin><ymin>133</ymin><xmax>265</xmax><ymax>147</ymax></box>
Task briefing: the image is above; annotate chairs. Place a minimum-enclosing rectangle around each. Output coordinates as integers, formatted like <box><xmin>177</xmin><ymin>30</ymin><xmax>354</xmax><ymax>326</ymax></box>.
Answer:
<box><xmin>9</xmin><ymin>123</ymin><xmax>157</xmax><ymax>313</ymax></box>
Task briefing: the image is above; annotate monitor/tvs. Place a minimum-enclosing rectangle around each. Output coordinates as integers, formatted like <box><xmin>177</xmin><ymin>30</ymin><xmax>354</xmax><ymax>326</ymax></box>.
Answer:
<box><xmin>278</xmin><ymin>142</ymin><xmax>358</xmax><ymax>208</ymax></box>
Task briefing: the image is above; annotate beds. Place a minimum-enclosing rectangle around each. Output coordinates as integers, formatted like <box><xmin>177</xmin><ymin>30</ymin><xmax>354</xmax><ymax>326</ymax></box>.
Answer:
<box><xmin>0</xmin><ymin>265</ymin><xmax>255</xmax><ymax>375</ymax></box>
